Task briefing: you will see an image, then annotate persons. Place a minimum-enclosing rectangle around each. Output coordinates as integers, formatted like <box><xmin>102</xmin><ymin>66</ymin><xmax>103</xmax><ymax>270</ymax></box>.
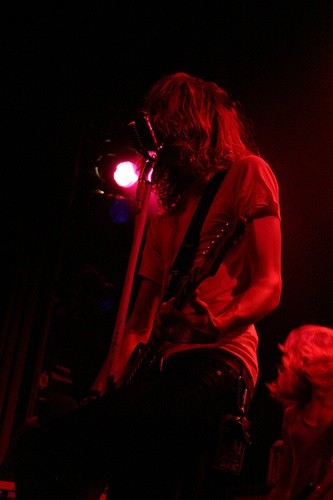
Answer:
<box><xmin>101</xmin><ymin>71</ymin><xmax>283</xmax><ymax>499</ymax></box>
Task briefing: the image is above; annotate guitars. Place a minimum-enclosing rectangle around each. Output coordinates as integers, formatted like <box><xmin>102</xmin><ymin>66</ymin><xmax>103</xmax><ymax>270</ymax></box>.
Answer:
<box><xmin>83</xmin><ymin>218</ymin><xmax>243</xmax><ymax>474</ymax></box>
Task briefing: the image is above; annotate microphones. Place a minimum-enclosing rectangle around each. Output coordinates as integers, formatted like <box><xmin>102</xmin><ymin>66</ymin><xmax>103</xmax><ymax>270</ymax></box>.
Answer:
<box><xmin>129</xmin><ymin>120</ymin><xmax>160</xmax><ymax>184</ymax></box>
